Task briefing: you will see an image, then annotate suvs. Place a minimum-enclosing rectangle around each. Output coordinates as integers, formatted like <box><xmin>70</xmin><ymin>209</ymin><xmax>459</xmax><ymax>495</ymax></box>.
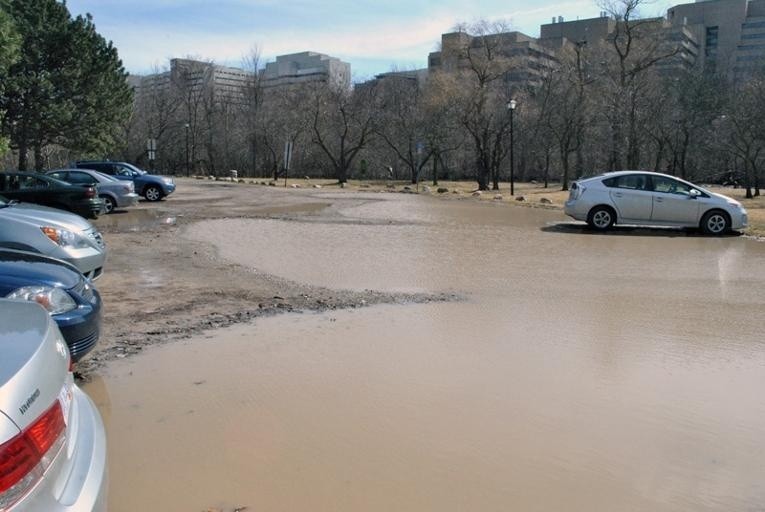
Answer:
<box><xmin>70</xmin><ymin>160</ymin><xmax>177</xmax><ymax>203</ymax></box>
<box><xmin>0</xmin><ymin>194</ymin><xmax>109</xmax><ymax>282</ymax></box>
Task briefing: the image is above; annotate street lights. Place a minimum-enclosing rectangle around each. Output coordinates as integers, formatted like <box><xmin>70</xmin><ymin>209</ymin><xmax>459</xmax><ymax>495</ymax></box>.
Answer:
<box><xmin>507</xmin><ymin>97</ymin><xmax>518</xmax><ymax>196</ymax></box>
<box><xmin>184</xmin><ymin>121</ymin><xmax>190</xmax><ymax>178</ymax></box>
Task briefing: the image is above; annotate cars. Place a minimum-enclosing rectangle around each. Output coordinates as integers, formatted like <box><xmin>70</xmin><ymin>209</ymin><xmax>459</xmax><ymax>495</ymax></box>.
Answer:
<box><xmin>0</xmin><ymin>171</ymin><xmax>103</xmax><ymax>221</ymax></box>
<box><xmin>0</xmin><ymin>246</ymin><xmax>104</xmax><ymax>370</ymax></box>
<box><xmin>24</xmin><ymin>166</ymin><xmax>139</xmax><ymax>217</ymax></box>
<box><xmin>563</xmin><ymin>169</ymin><xmax>751</xmax><ymax>237</ymax></box>
<box><xmin>0</xmin><ymin>294</ymin><xmax>113</xmax><ymax>512</ymax></box>
